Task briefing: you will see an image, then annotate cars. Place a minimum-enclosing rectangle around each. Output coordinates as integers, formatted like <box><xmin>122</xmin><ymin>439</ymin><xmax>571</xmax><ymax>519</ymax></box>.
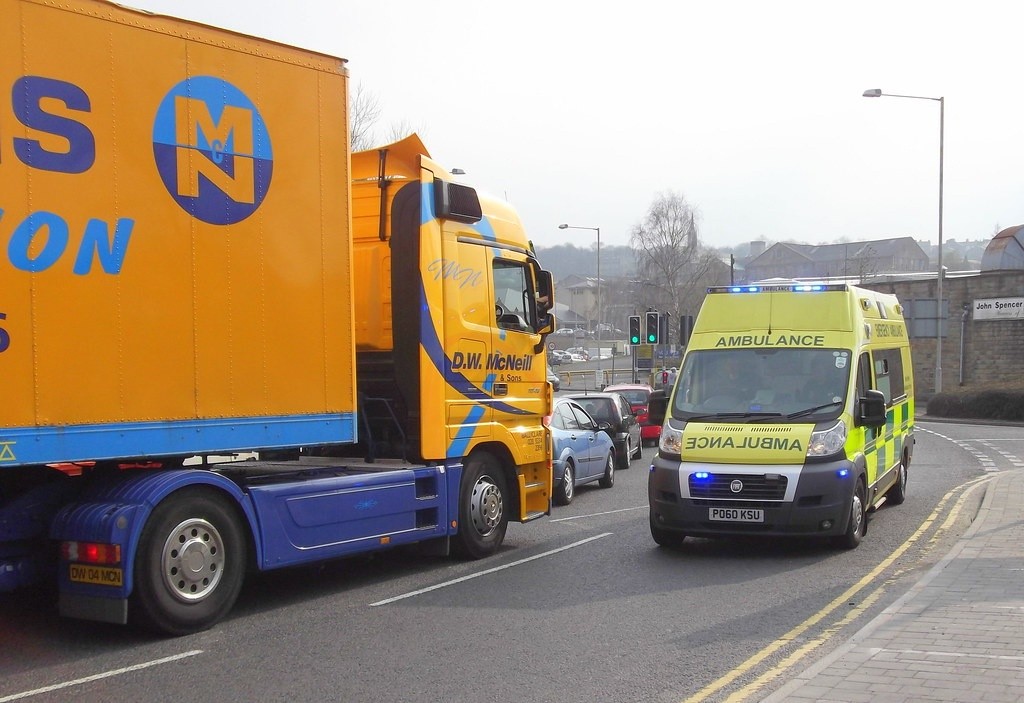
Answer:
<box><xmin>603</xmin><ymin>384</ymin><xmax>661</xmax><ymax>446</ymax></box>
<box><xmin>560</xmin><ymin>392</ymin><xmax>642</xmax><ymax>469</ymax></box>
<box><xmin>551</xmin><ymin>398</ymin><xmax>617</xmax><ymax>507</ymax></box>
<box><xmin>556</xmin><ymin>329</ymin><xmax>572</xmax><ymax>334</ymax></box>
<box><xmin>548</xmin><ymin>348</ymin><xmax>613</xmax><ymax>364</ymax></box>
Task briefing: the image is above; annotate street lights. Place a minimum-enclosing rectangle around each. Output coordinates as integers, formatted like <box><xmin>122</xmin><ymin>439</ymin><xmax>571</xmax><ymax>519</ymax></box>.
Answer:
<box><xmin>862</xmin><ymin>88</ymin><xmax>944</xmax><ymax>394</ymax></box>
<box><xmin>557</xmin><ymin>223</ymin><xmax>601</xmax><ymax>390</ymax></box>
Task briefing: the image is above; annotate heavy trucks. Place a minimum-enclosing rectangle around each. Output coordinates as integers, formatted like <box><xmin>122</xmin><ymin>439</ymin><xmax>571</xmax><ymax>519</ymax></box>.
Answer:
<box><xmin>0</xmin><ymin>0</ymin><xmax>560</xmax><ymax>639</ymax></box>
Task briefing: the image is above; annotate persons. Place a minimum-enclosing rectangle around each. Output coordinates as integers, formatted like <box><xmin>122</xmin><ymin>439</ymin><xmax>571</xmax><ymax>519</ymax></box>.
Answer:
<box><xmin>668</xmin><ymin>367</ymin><xmax>677</xmax><ymax>395</ymax></box>
<box><xmin>802</xmin><ymin>358</ymin><xmax>839</xmax><ymax>402</ymax></box>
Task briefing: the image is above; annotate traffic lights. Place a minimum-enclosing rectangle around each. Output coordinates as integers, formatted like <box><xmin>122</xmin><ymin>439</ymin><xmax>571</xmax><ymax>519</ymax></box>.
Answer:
<box><xmin>627</xmin><ymin>315</ymin><xmax>641</xmax><ymax>347</ymax></box>
<box><xmin>646</xmin><ymin>311</ymin><xmax>659</xmax><ymax>345</ymax></box>
<box><xmin>662</xmin><ymin>372</ymin><xmax>667</xmax><ymax>384</ymax></box>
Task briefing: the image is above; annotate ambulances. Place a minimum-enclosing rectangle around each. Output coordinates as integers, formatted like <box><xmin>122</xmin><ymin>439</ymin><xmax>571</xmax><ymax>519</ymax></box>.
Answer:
<box><xmin>647</xmin><ymin>282</ymin><xmax>915</xmax><ymax>549</ymax></box>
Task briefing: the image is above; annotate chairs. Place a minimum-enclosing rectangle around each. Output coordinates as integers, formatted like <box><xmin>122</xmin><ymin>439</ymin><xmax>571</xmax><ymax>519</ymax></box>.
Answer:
<box><xmin>586</xmin><ymin>404</ymin><xmax>609</xmax><ymax>418</ymax></box>
<box><xmin>636</xmin><ymin>392</ymin><xmax>646</xmax><ymax>401</ymax></box>
